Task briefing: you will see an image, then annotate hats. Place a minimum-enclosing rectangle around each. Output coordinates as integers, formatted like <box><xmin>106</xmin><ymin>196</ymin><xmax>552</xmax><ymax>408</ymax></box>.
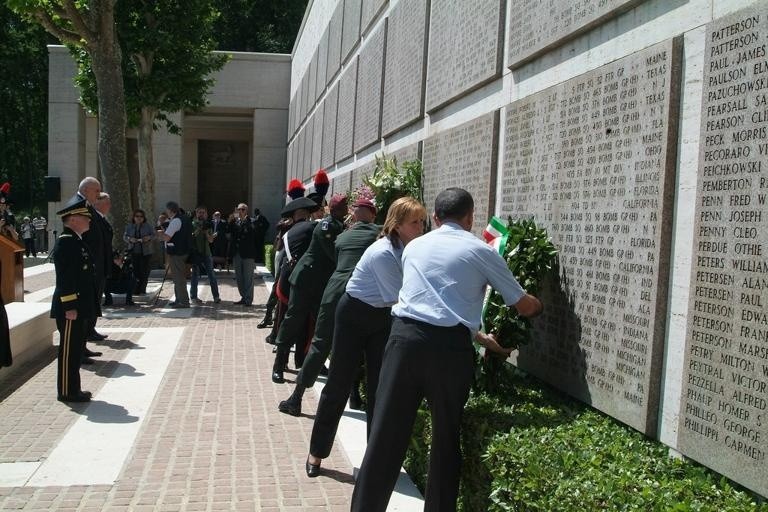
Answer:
<box><xmin>56</xmin><ymin>199</ymin><xmax>92</xmax><ymax>217</ymax></box>
<box><xmin>306</xmin><ymin>193</ymin><xmax>327</xmax><ymax>214</ymax></box>
<box><xmin>23</xmin><ymin>217</ymin><xmax>30</xmax><ymax>222</ymax></box>
<box><xmin>352</xmin><ymin>199</ymin><xmax>376</xmax><ymax>214</ymax></box>
<box><xmin>0</xmin><ymin>191</ymin><xmax>8</xmax><ymax>204</ymax></box>
<box><xmin>329</xmin><ymin>195</ymin><xmax>348</xmax><ymax>211</ymax></box>
<box><xmin>281</xmin><ymin>198</ymin><xmax>318</xmax><ymax>218</ymax></box>
<box><xmin>112</xmin><ymin>249</ymin><xmax>123</xmax><ymax>259</ymax></box>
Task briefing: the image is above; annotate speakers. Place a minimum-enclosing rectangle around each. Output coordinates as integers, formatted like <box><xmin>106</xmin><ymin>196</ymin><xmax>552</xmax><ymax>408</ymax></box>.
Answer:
<box><xmin>44</xmin><ymin>176</ymin><xmax>61</xmax><ymax>203</ymax></box>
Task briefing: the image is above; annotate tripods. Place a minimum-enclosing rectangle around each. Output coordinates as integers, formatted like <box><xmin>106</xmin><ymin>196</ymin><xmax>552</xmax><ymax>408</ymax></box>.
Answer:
<box><xmin>41</xmin><ymin>202</ymin><xmax>58</xmax><ymax>264</ymax></box>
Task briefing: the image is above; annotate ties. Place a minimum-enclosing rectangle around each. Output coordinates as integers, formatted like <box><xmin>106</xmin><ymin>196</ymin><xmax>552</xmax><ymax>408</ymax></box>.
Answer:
<box><xmin>216</xmin><ymin>220</ymin><xmax>218</xmax><ymax>232</ymax></box>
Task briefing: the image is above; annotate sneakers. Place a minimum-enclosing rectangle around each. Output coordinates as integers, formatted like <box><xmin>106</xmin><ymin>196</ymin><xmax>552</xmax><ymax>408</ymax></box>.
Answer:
<box><xmin>169</xmin><ymin>299</ymin><xmax>190</xmax><ymax>308</ymax></box>
<box><xmin>246</xmin><ymin>300</ymin><xmax>252</xmax><ymax>306</ymax></box>
<box><xmin>234</xmin><ymin>298</ymin><xmax>245</xmax><ymax>304</ymax></box>
<box><xmin>214</xmin><ymin>297</ymin><xmax>221</xmax><ymax>303</ymax></box>
<box><xmin>191</xmin><ymin>298</ymin><xmax>202</xmax><ymax>305</ymax></box>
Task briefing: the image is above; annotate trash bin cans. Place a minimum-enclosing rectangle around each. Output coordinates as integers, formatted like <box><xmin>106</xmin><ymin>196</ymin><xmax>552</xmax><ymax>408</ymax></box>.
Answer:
<box><xmin>1</xmin><ymin>235</ymin><xmax>26</xmax><ymax>303</ymax></box>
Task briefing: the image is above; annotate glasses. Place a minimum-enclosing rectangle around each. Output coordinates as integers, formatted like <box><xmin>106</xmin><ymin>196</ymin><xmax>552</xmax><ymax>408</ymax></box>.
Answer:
<box><xmin>199</xmin><ymin>211</ymin><xmax>205</xmax><ymax>214</ymax></box>
<box><xmin>135</xmin><ymin>215</ymin><xmax>143</xmax><ymax>217</ymax></box>
<box><xmin>237</xmin><ymin>209</ymin><xmax>245</xmax><ymax>211</ymax></box>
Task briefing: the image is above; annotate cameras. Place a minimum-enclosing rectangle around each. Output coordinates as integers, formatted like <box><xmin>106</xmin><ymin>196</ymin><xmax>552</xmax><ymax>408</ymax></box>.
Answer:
<box><xmin>230</xmin><ymin>212</ymin><xmax>240</xmax><ymax>219</ymax></box>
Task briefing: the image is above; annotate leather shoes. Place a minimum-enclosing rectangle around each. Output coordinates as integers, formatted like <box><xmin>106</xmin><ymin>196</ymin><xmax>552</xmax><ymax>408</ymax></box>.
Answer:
<box><xmin>81</xmin><ymin>357</ymin><xmax>96</xmax><ymax>365</ymax></box>
<box><xmin>272</xmin><ymin>368</ymin><xmax>285</xmax><ymax>383</ymax></box>
<box><xmin>266</xmin><ymin>336</ymin><xmax>275</xmax><ymax>344</ymax></box>
<box><xmin>88</xmin><ymin>333</ymin><xmax>108</xmax><ymax>341</ymax></box>
<box><xmin>126</xmin><ymin>300</ymin><xmax>134</xmax><ymax>305</ymax></box>
<box><xmin>279</xmin><ymin>396</ymin><xmax>301</xmax><ymax>417</ymax></box>
<box><xmin>81</xmin><ymin>348</ymin><xmax>102</xmax><ymax>357</ymax></box>
<box><xmin>257</xmin><ymin>319</ymin><xmax>272</xmax><ymax>328</ymax></box>
<box><xmin>221</xmin><ymin>264</ymin><xmax>225</xmax><ymax>269</ymax></box>
<box><xmin>104</xmin><ymin>300</ymin><xmax>112</xmax><ymax>308</ymax></box>
<box><xmin>306</xmin><ymin>452</ymin><xmax>321</xmax><ymax>477</ymax></box>
<box><xmin>213</xmin><ymin>263</ymin><xmax>217</xmax><ymax>268</ymax></box>
<box><xmin>58</xmin><ymin>391</ymin><xmax>91</xmax><ymax>402</ymax></box>
<box><xmin>320</xmin><ymin>365</ymin><xmax>328</xmax><ymax>375</ymax></box>
<box><xmin>351</xmin><ymin>393</ymin><xmax>366</xmax><ymax>411</ymax></box>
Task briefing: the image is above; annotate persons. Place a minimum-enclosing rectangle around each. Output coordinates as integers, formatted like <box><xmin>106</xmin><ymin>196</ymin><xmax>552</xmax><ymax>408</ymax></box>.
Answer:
<box><xmin>346</xmin><ymin>187</ymin><xmax>543</xmax><ymax>511</ymax></box>
<box><xmin>0</xmin><ymin>181</ymin><xmax>19</xmax><ymax>241</ymax></box>
<box><xmin>48</xmin><ymin>199</ymin><xmax>102</xmax><ymax>402</ymax></box>
<box><xmin>277</xmin><ymin>197</ymin><xmax>383</xmax><ymax>418</ymax></box>
<box><xmin>101</xmin><ymin>249</ymin><xmax>140</xmax><ymax>308</ymax></box>
<box><xmin>272</xmin><ymin>195</ymin><xmax>350</xmax><ymax>385</ymax></box>
<box><xmin>304</xmin><ymin>197</ymin><xmax>430</xmax><ymax>481</ymax></box>
<box><xmin>88</xmin><ymin>191</ymin><xmax>113</xmax><ymax>344</ymax></box>
<box><xmin>257</xmin><ymin>194</ymin><xmax>329</xmax><ymax>346</ymax></box>
<box><xmin>0</xmin><ymin>258</ymin><xmax>12</xmax><ymax>370</ymax></box>
<box><xmin>20</xmin><ymin>216</ymin><xmax>36</xmax><ymax>257</ymax></box>
<box><xmin>63</xmin><ymin>176</ymin><xmax>103</xmax><ymax>364</ymax></box>
<box><xmin>32</xmin><ymin>211</ymin><xmax>48</xmax><ymax>253</ymax></box>
<box><xmin>122</xmin><ymin>200</ymin><xmax>269</xmax><ymax>309</ymax></box>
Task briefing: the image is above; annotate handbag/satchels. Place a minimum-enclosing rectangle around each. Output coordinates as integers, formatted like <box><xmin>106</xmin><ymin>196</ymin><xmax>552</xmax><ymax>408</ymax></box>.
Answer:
<box><xmin>274</xmin><ymin>230</ymin><xmax>283</xmax><ymax>251</ymax></box>
<box><xmin>185</xmin><ymin>234</ymin><xmax>200</xmax><ymax>264</ymax></box>
